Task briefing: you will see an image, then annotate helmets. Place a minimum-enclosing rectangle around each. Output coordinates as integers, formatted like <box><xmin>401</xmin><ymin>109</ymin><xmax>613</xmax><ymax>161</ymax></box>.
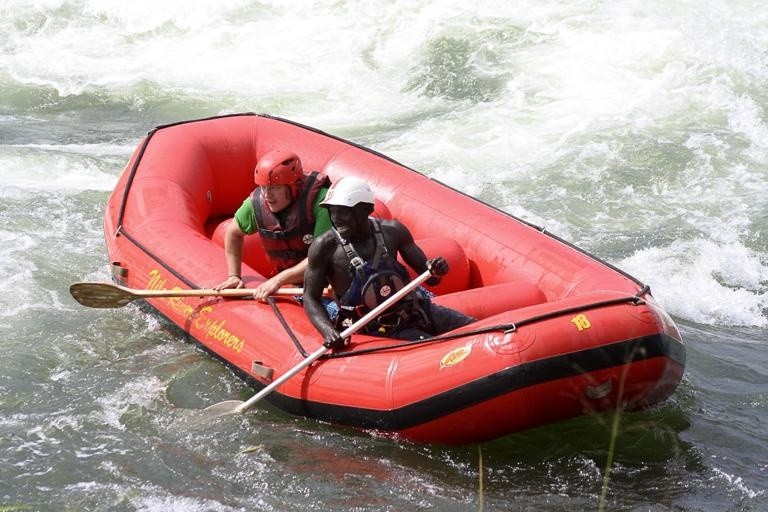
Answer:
<box><xmin>254</xmin><ymin>149</ymin><xmax>303</xmax><ymax>199</ymax></box>
<box><xmin>318</xmin><ymin>176</ymin><xmax>375</xmax><ymax>208</ymax></box>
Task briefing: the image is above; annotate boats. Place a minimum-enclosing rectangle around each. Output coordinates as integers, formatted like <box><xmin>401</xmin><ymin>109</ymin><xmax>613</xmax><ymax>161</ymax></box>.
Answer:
<box><xmin>104</xmin><ymin>112</ymin><xmax>687</xmax><ymax>446</ymax></box>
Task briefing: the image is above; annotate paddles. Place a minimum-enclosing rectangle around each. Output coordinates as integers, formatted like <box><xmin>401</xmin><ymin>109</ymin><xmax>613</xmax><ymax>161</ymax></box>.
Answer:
<box><xmin>70</xmin><ymin>282</ymin><xmax>333</xmax><ymax>308</ymax></box>
<box><xmin>204</xmin><ymin>272</ymin><xmax>433</xmax><ymax>413</ymax></box>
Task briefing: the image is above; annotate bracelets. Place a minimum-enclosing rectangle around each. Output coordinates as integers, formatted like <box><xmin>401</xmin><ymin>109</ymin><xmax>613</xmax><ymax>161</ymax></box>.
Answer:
<box><xmin>229</xmin><ymin>275</ymin><xmax>242</xmax><ymax>280</ymax></box>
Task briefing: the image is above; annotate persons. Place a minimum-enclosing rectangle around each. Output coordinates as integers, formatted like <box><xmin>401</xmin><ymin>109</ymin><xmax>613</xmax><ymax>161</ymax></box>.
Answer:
<box><xmin>303</xmin><ymin>176</ymin><xmax>479</xmax><ymax>349</ymax></box>
<box><xmin>212</xmin><ymin>149</ymin><xmax>437</xmax><ymax>323</ymax></box>
